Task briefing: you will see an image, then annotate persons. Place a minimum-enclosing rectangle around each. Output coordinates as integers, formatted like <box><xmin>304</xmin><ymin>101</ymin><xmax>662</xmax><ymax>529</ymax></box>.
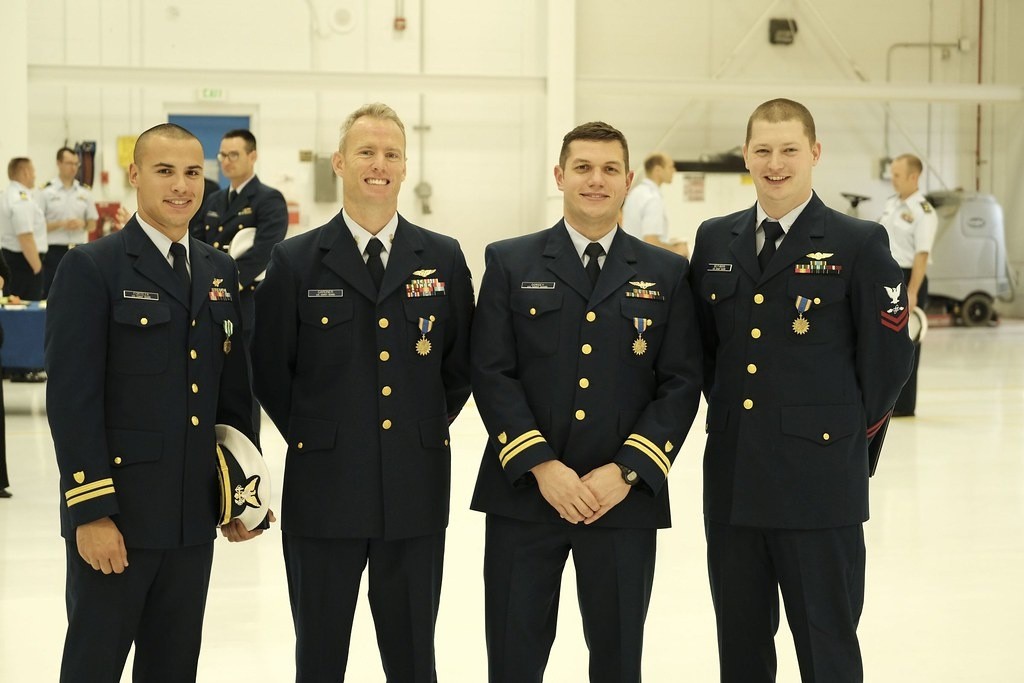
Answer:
<box><xmin>0</xmin><ymin>250</ymin><xmax>12</xmax><ymax>498</ymax></box>
<box><xmin>469</xmin><ymin>122</ymin><xmax>700</xmax><ymax>683</ymax></box>
<box><xmin>0</xmin><ymin>148</ymin><xmax>48</xmax><ymax>382</ymax></box>
<box><xmin>193</xmin><ymin>130</ymin><xmax>288</xmax><ymax>313</ymax></box>
<box><xmin>187</xmin><ymin>178</ymin><xmax>220</xmax><ymax>228</ymax></box>
<box><xmin>622</xmin><ymin>152</ymin><xmax>688</xmax><ymax>259</ymax></box>
<box><xmin>880</xmin><ymin>155</ymin><xmax>936</xmax><ymax>416</ymax></box>
<box><xmin>691</xmin><ymin>99</ymin><xmax>915</xmax><ymax>683</ymax></box>
<box><xmin>39</xmin><ymin>147</ymin><xmax>98</xmax><ymax>270</ymax></box>
<box><xmin>254</xmin><ymin>102</ymin><xmax>476</xmax><ymax>683</ymax></box>
<box><xmin>44</xmin><ymin>122</ymin><xmax>277</xmax><ymax>683</ymax></box>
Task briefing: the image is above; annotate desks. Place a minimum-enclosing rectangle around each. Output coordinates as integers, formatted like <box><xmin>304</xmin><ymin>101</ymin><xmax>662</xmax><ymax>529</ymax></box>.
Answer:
<box><xmin>0</xmin><ymin>301</ymin><xmax>47</xmax><ymax>369</ymax></box>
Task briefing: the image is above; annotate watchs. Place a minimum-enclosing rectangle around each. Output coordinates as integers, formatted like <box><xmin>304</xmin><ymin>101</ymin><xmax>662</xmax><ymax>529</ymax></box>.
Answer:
<box><xmin>615</xmin><ymin>461</ymin><xmax>640</xmax><ymax>485</ymax></box>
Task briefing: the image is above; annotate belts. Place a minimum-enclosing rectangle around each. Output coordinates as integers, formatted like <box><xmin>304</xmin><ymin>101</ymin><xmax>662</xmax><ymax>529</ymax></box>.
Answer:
<box><xmin>49</xmin><ymin>244</ymin><xmax>76</xmax><ymax>249</ymax></box>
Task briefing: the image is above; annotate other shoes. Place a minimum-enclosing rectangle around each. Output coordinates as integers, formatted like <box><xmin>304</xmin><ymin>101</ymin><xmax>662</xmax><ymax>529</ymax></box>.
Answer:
<box><xmin>10</xmin><ymin>372</ymin><xmax>45</xmax><ymax>383</ymax></box>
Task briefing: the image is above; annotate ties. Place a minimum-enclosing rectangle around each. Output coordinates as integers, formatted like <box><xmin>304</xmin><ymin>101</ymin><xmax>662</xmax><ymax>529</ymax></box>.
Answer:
<box><xmin>365</xmin><ymin>236</ymin><xmax>386</xmax><ymax>292</ymax></box>
<box><xmin>584</xmin><ymin>243</ymin><xmax>603</xmax><ymax>286</ymax></box>
<box><xmin>169</xmin><ymin>244</ymin><xmax>188</xmax><ymax>283</ymax></box>
<box><xmin>757</xmin><ymin>220</ymin><xmax>783</xmax><ymax>272</ymax></box>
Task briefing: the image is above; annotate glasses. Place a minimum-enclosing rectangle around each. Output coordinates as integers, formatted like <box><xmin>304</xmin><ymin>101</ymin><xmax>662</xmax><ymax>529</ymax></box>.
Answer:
<box><xmin>217</xmin><ymin>153</ymin><xmax>240</xmax><ymax>163</ymax></box>
<box><xmin>65</xmin><ymin>161</ymin><xmax>81</xmax><ymax>168</ymax></box>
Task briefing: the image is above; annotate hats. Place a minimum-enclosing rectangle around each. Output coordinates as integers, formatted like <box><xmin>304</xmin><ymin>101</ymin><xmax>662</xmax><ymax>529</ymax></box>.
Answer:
<box><xmin>214</xmin><ymin>420</ymin><xmax>271</xmax><ymax>534</ymax></box>
<box><xmin>908</xmin><ymin>305</ymin><xmax>927</xmax><ymax>343</ymax></box>
<box><xmin>229</xmin><ymin>228</ymin><xmax>267</xmax><ymax>280</ymax></box>
